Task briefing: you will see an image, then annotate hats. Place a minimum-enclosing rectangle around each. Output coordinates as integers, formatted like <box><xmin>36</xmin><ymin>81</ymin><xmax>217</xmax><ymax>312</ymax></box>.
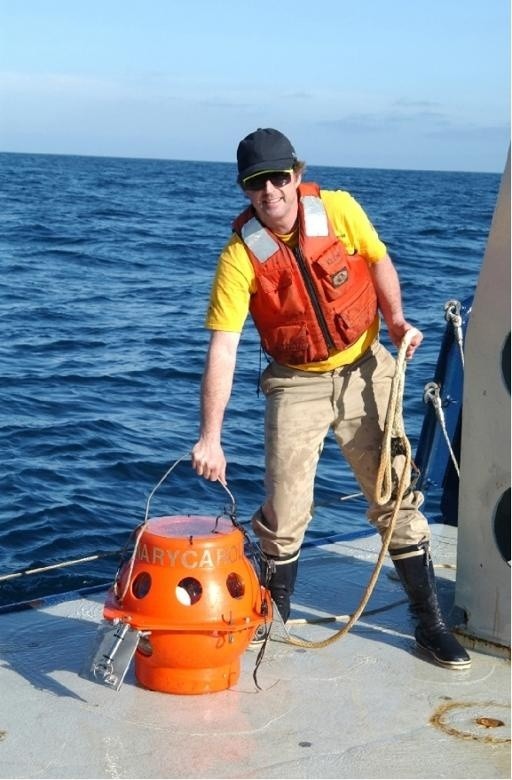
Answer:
<box><xmin>236</xmin><ymin>128</ymin><xmax>298</xmax><ymax>184</ymax></box>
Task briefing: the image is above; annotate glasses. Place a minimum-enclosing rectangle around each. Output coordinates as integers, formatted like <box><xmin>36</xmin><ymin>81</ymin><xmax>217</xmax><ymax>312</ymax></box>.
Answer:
<box><xmin>243</xmin><ymin>172</ymin><xmax>291</xmax><ymax>190</ymax></box>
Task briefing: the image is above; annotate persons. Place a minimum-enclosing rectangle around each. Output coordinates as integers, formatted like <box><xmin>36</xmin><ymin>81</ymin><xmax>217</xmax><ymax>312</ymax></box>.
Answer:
<box><xmin>191</xmin><ymin>123</ymin><xmax>472</xmax><ymax>672</ymax></box>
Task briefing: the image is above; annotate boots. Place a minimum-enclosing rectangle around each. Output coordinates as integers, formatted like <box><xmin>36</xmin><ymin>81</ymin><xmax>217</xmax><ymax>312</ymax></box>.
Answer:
<box><xmin>385</xmin><ymin>540</ymin><xmax>472</xmax><ymax>670</ymax></box>
<box><xmin>250</xmin><ymin>549</ymin><xmax>300</xmax><ymax>643</ymax></box>
